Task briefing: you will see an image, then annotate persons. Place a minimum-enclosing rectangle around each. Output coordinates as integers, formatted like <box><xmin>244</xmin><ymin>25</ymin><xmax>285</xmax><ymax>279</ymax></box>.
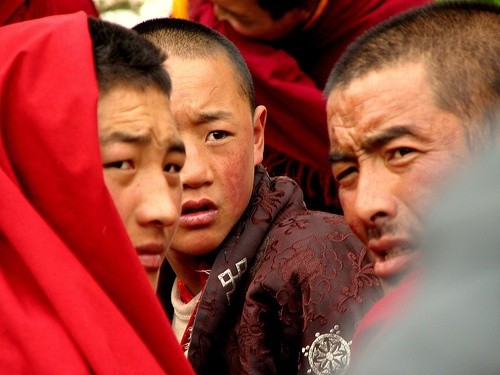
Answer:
<box><xmin>0</xmin><ymin>12</ymin><xmax>187</xmax><ymax>375</ymax></box>
<box><xmin>0</xmin><ymin>0</ymin><xmax>143</xmax><ymax>28</ymax></box>
<box><xmin>325</xmin><ymin>0</ymin><xmax>500</xmax><ymax>363</ymax></box>
<box><xmin>133</xmin><ymin>18</ymin><xmax>383</xmax><ymax>374</ymax></box>
<box><xmin>213</xmin><ymin>0</ymin><xmax>323</xmax><ymax>41</ymax></box>
<box><xmin>170</xmin><ymin>1</ymin><xmax>435</xmax><ymax>212</ymax></box>
